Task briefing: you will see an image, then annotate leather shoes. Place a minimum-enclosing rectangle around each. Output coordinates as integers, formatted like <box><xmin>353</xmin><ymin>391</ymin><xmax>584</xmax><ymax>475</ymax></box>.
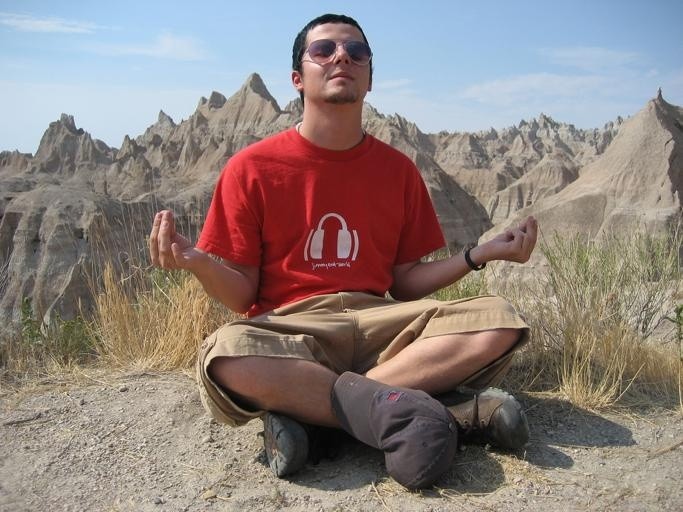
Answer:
<box><xmin>263</xmin><ymin>411</ymin><xmax>315</xmax><ymax>478</ymax></box>
<box><xmin>431</xmin><ymin>385</ymin><xmax>530</xmax><ymax>452</ymax></box>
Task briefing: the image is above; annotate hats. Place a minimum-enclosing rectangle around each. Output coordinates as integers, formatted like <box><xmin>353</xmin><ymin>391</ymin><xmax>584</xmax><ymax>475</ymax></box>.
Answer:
<box><xmin>331</xmin><ymin>370</ymin><xmax>458</xmax><ymax>489</ymax></box>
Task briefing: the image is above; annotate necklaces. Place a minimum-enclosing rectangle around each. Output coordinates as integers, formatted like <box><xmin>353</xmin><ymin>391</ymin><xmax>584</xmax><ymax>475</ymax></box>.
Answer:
<box><xmin>295</xmin><ymin>121</ymin><xmax>365</xmax><ymax>136</ymax></box>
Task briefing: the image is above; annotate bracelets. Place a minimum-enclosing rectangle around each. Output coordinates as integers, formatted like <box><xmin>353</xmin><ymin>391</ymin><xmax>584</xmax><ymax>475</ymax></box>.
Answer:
<box><xmin>464</xmin><ymin>247</ymin><xmax>486</xmax><ymax>271</ymax></box>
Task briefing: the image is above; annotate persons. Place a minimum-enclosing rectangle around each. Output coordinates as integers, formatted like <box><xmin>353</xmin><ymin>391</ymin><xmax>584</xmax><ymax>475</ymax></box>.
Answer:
<box><xmin>147</xmin><ymin>14</ymin><xmax>538</xmax><ymax>481</ymax></box>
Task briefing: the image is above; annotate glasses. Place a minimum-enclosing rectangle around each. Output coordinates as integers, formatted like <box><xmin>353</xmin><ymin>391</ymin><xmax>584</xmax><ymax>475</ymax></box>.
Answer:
<box><xmin>299</xmin><ymin>39</ymin><xmax>373</xmax><ymax>66</ymax></box>
